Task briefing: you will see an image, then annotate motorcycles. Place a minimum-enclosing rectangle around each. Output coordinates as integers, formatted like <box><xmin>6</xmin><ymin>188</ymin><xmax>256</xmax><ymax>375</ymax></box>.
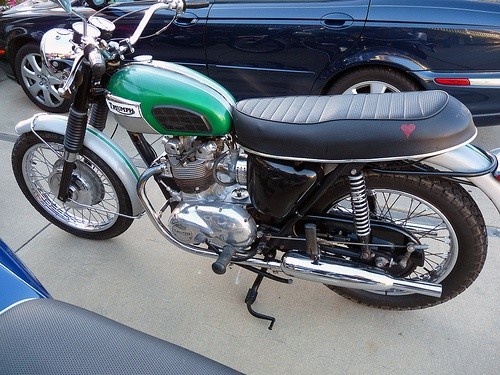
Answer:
<box><xmin>11</xmin><ymin>1</ymin><xmax>499</xmax><ymax>330</ymax></box>
<box><xmin>0</xmin><ymin>237</ymin><xmax>250</xmax><ymax>375</ymax></box>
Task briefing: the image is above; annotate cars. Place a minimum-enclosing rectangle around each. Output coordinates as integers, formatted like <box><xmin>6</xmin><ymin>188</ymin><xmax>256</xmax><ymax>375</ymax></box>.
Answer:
<box><xmin>0</xmin><ymin>1</ymin><xmax>499</xmax><ymax>127</ymax></box>
